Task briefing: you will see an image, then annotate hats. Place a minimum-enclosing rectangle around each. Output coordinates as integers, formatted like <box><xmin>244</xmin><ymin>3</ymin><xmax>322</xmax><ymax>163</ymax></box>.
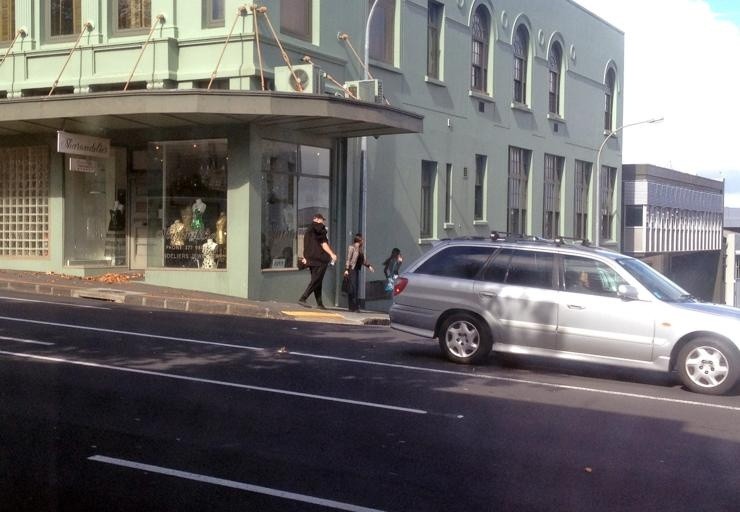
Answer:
<box><xmin>314</xmin><ymin>213</ymin><xmax>326</xmax><ymax>221</ymax></box>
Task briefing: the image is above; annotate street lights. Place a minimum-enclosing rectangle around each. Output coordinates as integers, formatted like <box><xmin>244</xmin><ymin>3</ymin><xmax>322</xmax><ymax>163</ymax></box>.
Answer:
<box><xmin>593</xmin><ymin>115</ymin><xmax>666</xmax><ymax>247</ymax></box>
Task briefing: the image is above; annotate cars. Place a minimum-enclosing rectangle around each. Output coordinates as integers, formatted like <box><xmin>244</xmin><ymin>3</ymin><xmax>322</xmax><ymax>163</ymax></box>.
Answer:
<box><xmin>264</xmin><ymin>224</ymin><xmax>308</xmax><ymax>269</ymax></box>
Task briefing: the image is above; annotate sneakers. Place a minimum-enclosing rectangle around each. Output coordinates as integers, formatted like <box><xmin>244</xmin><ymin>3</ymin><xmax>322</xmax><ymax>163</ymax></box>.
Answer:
<box><xmin>316</xmin><ymin>304</ymin><xmax>328</xmax><ymax>310</ymax></box>
<box><xmin>298</xmin><ymin>300</ymin><xmax>312</xmax><ymax>308</ymax></box>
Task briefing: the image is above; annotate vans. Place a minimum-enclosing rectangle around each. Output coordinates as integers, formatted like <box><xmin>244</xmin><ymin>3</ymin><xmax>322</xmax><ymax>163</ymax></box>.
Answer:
<box><xmin>390</xmin><ymin>232</ymin><xmax>740</xmax><ymax>395</ymax></box>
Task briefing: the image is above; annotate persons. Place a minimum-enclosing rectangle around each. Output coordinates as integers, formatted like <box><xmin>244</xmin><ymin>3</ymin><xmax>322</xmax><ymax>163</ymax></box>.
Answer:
<box><xmin>343</xmin><ymin>233</ymin><xmax>374</xmax><ymax>313</ymax></box>
<box><xmin>168</xmin><ymin>198</ymin><xmax>227</xmax><ymax>269</ymax></box>
<box><xmin>384</xmin><ymin>248</ymin><xmax>403</xmax><ymax>282</ymax></box>
<box><xmin>108</xmin><ymin>200</ymin><xmax>125</xmax><ymax>231</ymax></box>
<box><xmin>297</xmin><ymin>213</ymin><xmax>337</xmax><ymax>310</ymax></box>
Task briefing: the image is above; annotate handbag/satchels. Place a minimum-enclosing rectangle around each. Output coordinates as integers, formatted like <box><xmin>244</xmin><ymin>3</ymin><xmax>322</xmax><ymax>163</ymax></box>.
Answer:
<box><xmin>341</xmin><ymin>276</ymin><xmax>351</xmax><ymax>293</ymax></box>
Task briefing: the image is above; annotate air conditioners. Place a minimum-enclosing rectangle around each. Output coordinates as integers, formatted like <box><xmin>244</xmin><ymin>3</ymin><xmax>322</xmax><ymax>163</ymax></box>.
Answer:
<box><xmin>344</xmin><ymin>80</ymin><xmax>383</xmax><ymax>103</ymax></box>
<box><xmin>275</xmin><ymin>64</ymin><xmax>321</xmax><ymax>94</ymax></box>
